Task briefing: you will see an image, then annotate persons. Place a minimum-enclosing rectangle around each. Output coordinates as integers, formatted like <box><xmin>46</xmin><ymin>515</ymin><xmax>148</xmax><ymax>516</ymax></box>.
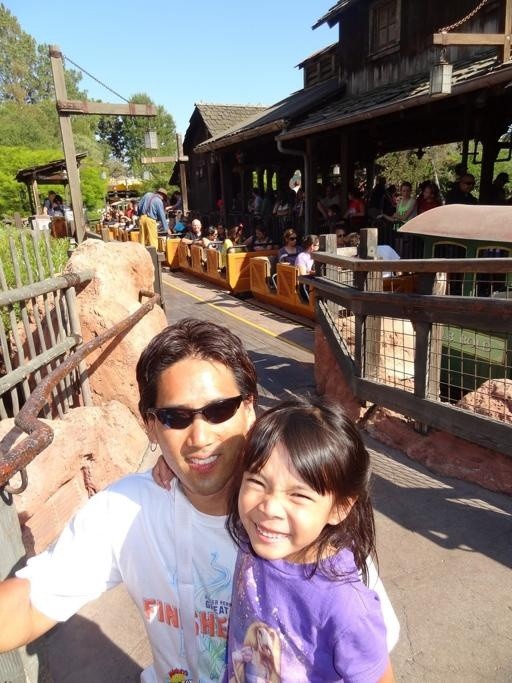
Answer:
<box><xmin>150</xmin><ymin>385</ymin><xmax>399</xmax><ymax>683</ymax></box>
<box><xmin>0</xmin><ymin>313</ymin><xmax>399</xmax><ymax>683</ymax></box>
<box><xmin>42</xmin><ymin>190</ymin><xmax>72</xmax><ymax>221</ymax></box>
<box><xmin>103</xmin><ymin>171</ymin><xmax>511</xmax><ymax>298</ymax></box>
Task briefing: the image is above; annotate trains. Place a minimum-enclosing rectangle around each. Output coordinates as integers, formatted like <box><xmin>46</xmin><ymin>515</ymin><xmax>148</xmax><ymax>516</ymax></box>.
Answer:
<box><xmin>96</xmin><ymin>203</ymin><xmax>511</xmax><ymax>404</ymax></box>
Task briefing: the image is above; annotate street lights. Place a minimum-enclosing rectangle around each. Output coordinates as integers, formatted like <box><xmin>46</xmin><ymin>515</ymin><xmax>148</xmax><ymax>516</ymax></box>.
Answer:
<box><xmin>423</xmin><ymin>0</ymin><xmax>512</xmax><ymax>98</ymax></box>
<box><xmin>141</xmin><ymin>132</ymin><xmax>190</xmax><ymax>217</ymax></box>
<box><xmin>50</xmin><ymin>44</ymin><xmax>158</xmax><ymax>247</ymax></box>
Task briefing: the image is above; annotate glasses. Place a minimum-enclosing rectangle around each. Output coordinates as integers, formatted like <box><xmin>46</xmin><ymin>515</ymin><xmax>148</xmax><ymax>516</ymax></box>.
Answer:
<box><xmin>289</xmin><ymin>237</ymin><xmax>296</xmax><ymax>241</ymax></box>
<box><xmin>147</xmin><ymin>396</ymin><xmax>247</xmax><ymax>429</ymax></box>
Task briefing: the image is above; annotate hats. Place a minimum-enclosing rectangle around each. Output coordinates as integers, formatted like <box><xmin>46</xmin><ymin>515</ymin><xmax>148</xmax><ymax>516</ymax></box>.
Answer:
<box><xmin>157</xmin><ymin>187</ymin><xmax>168</xmax><ymax>197</ymax></box>
